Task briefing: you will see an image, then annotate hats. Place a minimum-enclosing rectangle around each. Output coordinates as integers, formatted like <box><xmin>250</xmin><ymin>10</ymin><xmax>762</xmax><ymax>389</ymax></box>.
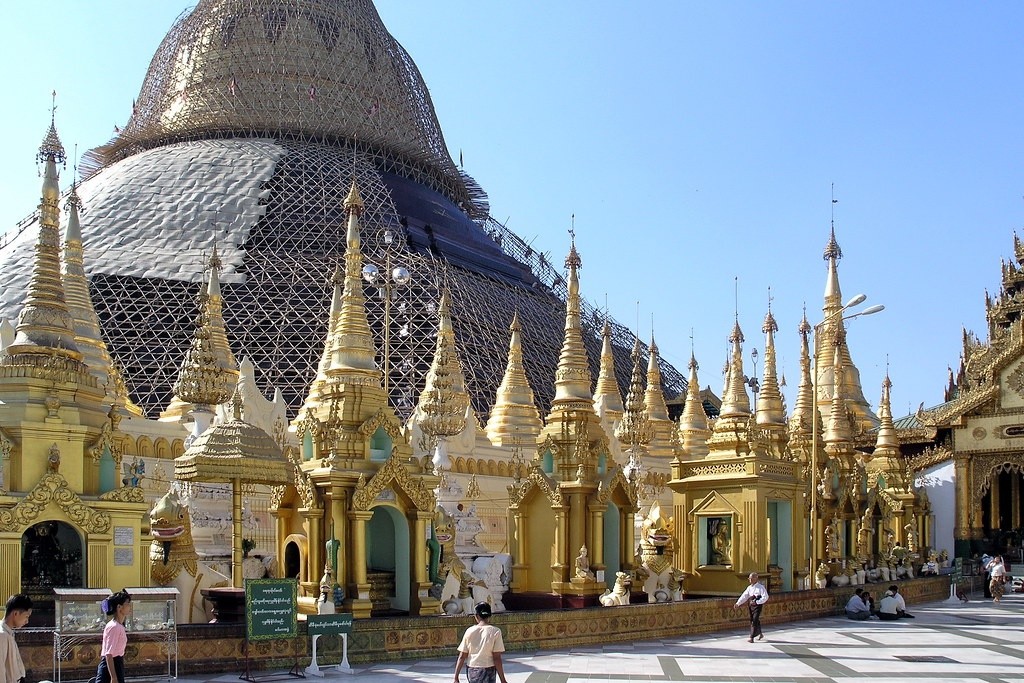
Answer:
<box><xmin>885</xmin><ymin>590</ymin><xmax>893</xmax><ymax>596</ymax></box>
<box><xmin>982</xmin><ymin>553</ymin><xmax>988</xmax><ymax>558</ymax></box>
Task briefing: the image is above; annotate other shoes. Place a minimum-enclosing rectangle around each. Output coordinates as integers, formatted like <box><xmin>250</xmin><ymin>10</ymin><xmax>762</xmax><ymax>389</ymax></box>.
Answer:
<box><xmin>869</xmin><ymin>615</ymin><xmax>879</xmax><ymax>619</ymax></box>
<box><xmin>904</xmin><ymin>613</ymin><xmax>915</xmax><ymax>618</ymax></box>
<box><xmin>748</xmin><ymin>640</ymin><xmax>754</xmax><ymax>642</ymax></box>
<box><xmin>758</xmin><ymin>635</ymin><xmax>763</xmax><ymax>640</ymax></box>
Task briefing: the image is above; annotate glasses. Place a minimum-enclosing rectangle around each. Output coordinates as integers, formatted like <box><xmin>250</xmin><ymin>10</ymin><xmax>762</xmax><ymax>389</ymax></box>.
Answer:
<box><xmin>747</xmin><ymin>578</ymin><xmax>756</xmax><ymax>580</ymax></box>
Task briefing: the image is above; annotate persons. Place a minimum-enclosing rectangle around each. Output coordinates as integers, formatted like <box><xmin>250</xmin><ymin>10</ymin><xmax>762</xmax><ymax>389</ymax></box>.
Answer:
<box><xmin>734</xmin><ymin>573</ymin><xmax>769</xmax><ymax>643</ymax></box>
<box><xmin>711</xmin><ymin>520</ymin><xmax>730</xmax><ymax>564</ymax></box>
<box><xmin>575</xmin><ymin>544</ymin><xmax>595</xmax><ymax>578</ymax></box>
<box><xmin>980</xmin><ymin>553</ymin><xmax>1006</xmax><ymax>603</ymax></box>
<box><xmin>0</xmin><ymin>593</ymin><xmax>33</xmax><ymax>683</ymax></box>
<box><xmin>845</xmin><ymin>585</ymin><xmax>905</xmax><ymax>620</ymax></box>
<box><xmin>893</xmin><ymin>542</ymin><xmax>907</xmax><ymax>566</ymax></box>
<box><xmin>95</xmin><ymin>588</ymin><xmax>132</xmax><ymax>683</ymax></box>
<box><xmin>453</xmin><ymin>601</ymin><xmax>508</xmax><ymax>683</ymax></box>
